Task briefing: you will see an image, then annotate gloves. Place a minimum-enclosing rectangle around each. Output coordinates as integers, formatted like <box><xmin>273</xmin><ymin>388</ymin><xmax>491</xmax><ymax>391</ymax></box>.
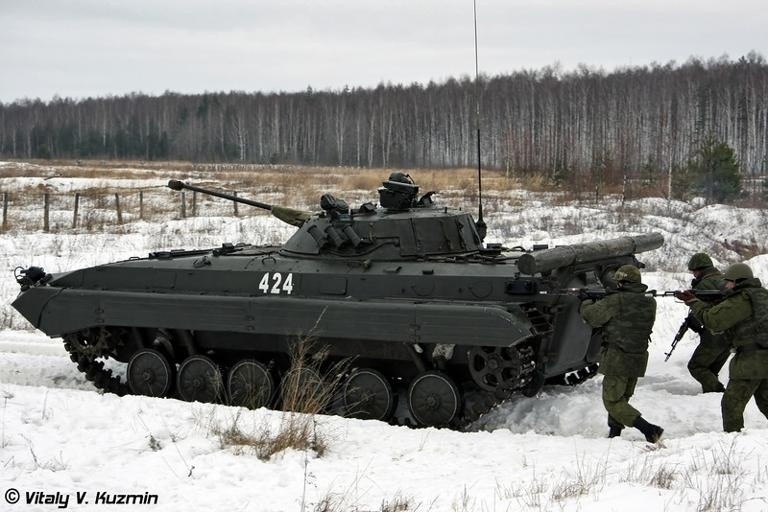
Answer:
<box><xmin>577</xmin><ymin>290</ymin><xmax>591</xmax><ymax>301</ymax></box>
<box><xmin>674</xmin><ymin>289</ymin><xmax>696</xmax><ymax>302</ymax></box>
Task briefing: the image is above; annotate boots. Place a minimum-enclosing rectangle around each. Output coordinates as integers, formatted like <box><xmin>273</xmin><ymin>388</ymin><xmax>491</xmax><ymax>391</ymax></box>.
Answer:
<box><xmin>633</xmin><ymin>415</ymin><xmax>664</xmax><ymax>444</ymax></box>
<box><xmin>607</xmin><ymin>422</ymin><xmax>625</xmax><ymax>439</ymax></box>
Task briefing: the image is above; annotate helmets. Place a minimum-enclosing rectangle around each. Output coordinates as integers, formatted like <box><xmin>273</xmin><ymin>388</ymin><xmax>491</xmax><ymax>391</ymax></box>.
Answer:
<box><xmin>613</xmin><ymin>264</ymin><xmax>642</xmax><ymax>284</ymax></box>
<box><xmin>725</xmin><ymin>263</ymin><xmax>754</xmax><ymax>281</ymax></box>
<box><xmin>688</xmin><ymin>253</ymin><xmax>713</xmax><ymax>272</ymax></box>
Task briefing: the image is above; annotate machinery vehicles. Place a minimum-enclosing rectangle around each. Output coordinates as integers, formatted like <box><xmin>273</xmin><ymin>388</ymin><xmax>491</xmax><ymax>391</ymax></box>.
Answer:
<box><xmin>12</xmin><ymin>172</ymin><xmax>664</xmax><ymax>430</ymax></box>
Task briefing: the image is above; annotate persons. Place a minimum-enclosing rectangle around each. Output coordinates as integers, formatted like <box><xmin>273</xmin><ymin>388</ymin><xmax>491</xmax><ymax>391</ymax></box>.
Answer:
<box><xmin>685</xmin><ymin>253</ymin><xmax>730</xmax><ymax>394</ymax></box>
<box><xmin>677</xmin><ymin>263</ymin><xmax>766</xmax><ymax>432</ymax></box>
<box><xmin>577</xmin><ymin>265</ymin><xmax>663</xmax><ymax>444</ymax></box>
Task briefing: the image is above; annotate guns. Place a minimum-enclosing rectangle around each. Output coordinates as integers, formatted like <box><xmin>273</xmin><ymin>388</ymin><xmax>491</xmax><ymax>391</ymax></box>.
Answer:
<box><xmin>664</xmin><ymin>310</ymin><xmax>702</xmax><ymax>361</ymax></box>
<box><xmin>558</xmin><ymin>289</ymin><xmax>621</xmax><ymax>303</ymax></box>
<box><xmin>653</xmin><ymin>290</ymin><xmax>724</xmax><ymax>303</ymax></box>
